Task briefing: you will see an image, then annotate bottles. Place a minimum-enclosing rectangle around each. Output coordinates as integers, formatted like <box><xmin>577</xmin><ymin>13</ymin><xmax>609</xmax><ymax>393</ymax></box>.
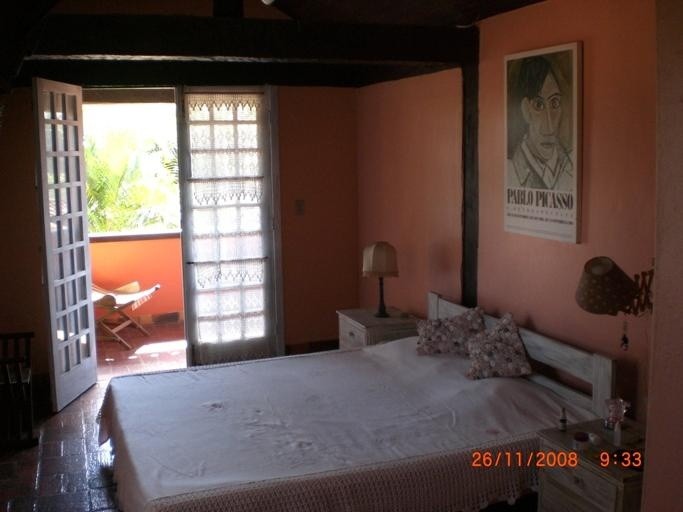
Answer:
<box><xmin>571</xmin><ymin>433</ymin><xmax>592</xmax><ymax>458</ymax></box>
<box><xmin>558</xmin><ymin>404</ymin><xmax>569</xmax><ymax>432</ymax></box>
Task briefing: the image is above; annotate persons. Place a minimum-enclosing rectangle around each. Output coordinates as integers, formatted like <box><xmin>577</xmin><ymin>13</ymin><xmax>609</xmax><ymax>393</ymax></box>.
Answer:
<box><xmin>506</xmin><ymin>56</ymin><xmax>574</xmax><ymax>192</ymax></box>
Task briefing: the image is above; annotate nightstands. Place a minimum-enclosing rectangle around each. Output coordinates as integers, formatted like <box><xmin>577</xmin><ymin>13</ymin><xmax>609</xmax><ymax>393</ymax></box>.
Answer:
<box><xmin>335</xmin><ymin>305</ymin><xmax>419</xmax><ymax>351</ymax></box>
<box><xmin>535</xmin><ymin>414</ymin><xmax>645</xmax><ymax>512</ymax></box>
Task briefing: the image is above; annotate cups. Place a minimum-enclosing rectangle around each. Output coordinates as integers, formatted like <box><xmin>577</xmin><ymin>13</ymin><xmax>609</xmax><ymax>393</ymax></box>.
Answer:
<box><xmin>602</xmin><ymin>394</ymin><xmax>632</xmax><ymax>430</ymax></box>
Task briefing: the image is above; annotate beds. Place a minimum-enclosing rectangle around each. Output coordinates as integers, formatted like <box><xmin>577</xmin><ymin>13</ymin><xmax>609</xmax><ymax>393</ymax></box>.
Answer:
<box><xmin>95</xmin><ymin>292</ymin><xmax>619</xmax><ymax>512</ymax></box>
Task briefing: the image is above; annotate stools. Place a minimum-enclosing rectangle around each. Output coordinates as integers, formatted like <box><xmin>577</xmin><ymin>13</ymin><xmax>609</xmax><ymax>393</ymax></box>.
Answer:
<box><xmin>0</xmin><ymin>330</ymin><xmax>40</xmax><ymax>453</ymax></box>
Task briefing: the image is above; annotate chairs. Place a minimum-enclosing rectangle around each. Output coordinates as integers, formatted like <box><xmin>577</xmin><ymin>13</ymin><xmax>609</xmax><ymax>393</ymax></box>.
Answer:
<box><xmin>90</xmin><ymin>280</ymin><xmax>160</xmax><ymax>351</ymax></box>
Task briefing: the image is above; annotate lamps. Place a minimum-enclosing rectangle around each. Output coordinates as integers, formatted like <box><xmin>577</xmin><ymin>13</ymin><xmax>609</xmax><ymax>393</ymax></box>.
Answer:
<box><xmin>361</xmin><ymin>240</ymin><xmax>399</xmax><ymax>318</ymax></box>
<box><xmin>574</xmin><ymin>254</ymin><xmax>655</xmax><ymax>350</ymax></box>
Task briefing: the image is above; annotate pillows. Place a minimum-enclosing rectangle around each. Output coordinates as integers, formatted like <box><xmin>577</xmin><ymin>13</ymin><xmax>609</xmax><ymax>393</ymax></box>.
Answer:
<box><xmin>463</xmin><ymin>310</ymin><xmax>535</xmax><ymax>381</ymax></box>
<box><xmin>415</xmin><ymin>305</ymin><xmax>488</xmax><ymax>355</ymax></box>
